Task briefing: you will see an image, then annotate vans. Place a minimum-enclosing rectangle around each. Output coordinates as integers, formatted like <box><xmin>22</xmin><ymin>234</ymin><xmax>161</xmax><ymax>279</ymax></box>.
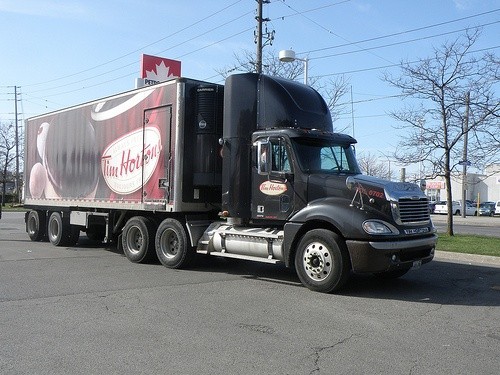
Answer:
<box><xmin>494</xmin><ymin>201</ymin><xmax>500</xmax><ymax>215</ymax></box>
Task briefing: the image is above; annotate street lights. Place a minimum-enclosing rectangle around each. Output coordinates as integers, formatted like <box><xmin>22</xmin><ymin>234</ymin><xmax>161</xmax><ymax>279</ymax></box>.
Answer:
<box><xmin>279</xmin><ymin>49</ymin><xmax>307</xmax><ymax>84</ymax></box>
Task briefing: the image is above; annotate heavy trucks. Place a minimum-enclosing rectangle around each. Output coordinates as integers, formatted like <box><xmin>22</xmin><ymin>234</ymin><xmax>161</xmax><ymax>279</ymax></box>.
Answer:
<box><xmin>19</xmin><ymin>73</ymin><xmax>439</xmax><ymax>294</ymax></box>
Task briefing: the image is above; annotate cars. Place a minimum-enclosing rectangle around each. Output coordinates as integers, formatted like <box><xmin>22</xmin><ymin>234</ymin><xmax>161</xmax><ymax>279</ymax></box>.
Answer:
<box><xmin>429</xmin><ymin>203</ymin><xmax>435</xmax><ymax>214</ymax></box>
<box><xmin>480</xmin><ymin>204</ymin><xmax>496</xmax><ymax>217</ymax></box>
<box><xmin>481</xmin><ymin>201</ymin><xmax>494</xmax><ymax>204</ymax></box>
<box><xmin>435</xmin><ymin>201</ymin><xmax>480</xmax><ymax>216</ymax></box>
<box><xmin>465</xmin><ymin>199</ymin><xmax>476</xmax><ymax>204</ymax></box>
